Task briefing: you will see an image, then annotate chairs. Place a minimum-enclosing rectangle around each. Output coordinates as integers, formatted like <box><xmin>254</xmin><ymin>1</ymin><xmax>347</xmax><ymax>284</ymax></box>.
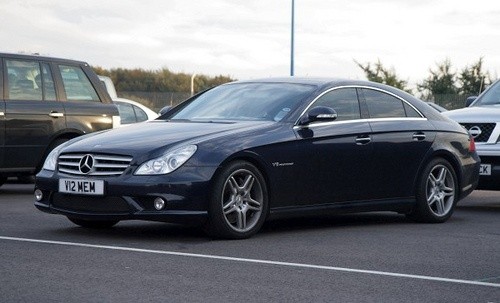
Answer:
<box><xmin>14</xmin><ymin>74</ymin><xmax>56</xmax><ymax>99</ymax></box>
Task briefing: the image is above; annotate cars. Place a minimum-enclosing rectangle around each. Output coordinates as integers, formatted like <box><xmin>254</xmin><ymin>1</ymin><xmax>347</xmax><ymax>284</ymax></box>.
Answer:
<box><xmin>32</xmin><ymin>77</ymin><xmax>483</xmax><ymax>244</ymax></box>
<box><xmin>112</xmin><ymin>99</ymin><xmax>161</xmax><ymax>130</ymax></box>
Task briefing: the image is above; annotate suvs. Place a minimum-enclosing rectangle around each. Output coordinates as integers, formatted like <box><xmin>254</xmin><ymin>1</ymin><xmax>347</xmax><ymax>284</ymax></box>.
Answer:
<box><xmin>443</xmin><ymin>79</ymin><xmax>500</xmax><ymax>188</ymax></box>
<box><xmin>0</xmin><ymin>52</ymin><xmax>120</xmax><ymax>189</ymax></box>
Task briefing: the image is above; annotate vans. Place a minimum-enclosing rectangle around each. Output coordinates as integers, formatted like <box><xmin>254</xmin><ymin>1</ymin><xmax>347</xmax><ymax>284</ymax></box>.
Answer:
<box><xmin>34</xmin><ymin>75</ymin><xmax>117</xmax><ymax>100</ymax></box>
